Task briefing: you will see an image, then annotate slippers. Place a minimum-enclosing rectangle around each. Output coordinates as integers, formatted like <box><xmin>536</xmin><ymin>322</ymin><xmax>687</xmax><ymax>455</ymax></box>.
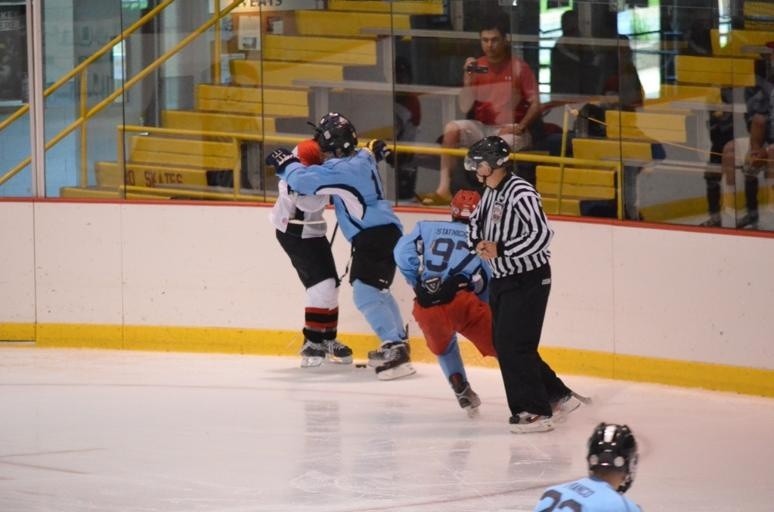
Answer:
<box><xmin>422</xmin><ymin>192</ymin><xmax>452</xmax><ymax>207</ymax></box>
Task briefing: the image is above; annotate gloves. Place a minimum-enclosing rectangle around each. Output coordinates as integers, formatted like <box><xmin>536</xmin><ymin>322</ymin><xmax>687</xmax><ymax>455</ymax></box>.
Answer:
<box><xmin>264</xmin><ymin>146</ymin><xmax>299</xmax><ymax>176</ymax></box>
<box><xmin>368</xmin><ymin>138</ymin><xmax>394</xmax><ymax>165</ymax></box>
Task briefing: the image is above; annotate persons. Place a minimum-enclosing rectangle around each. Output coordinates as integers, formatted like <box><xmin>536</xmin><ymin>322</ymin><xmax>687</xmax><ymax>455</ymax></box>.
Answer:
<box><xmin>264</xmin><ymin>111</ymin><xmax>413</xmax><ymax>374</ymax></box>
<box><xmin>549</xmin><ymin>10</ymin><xmax>591</xmax><ymax>94</ymax></box>
<box><xmin>588</xmin><ymin>35</ymin><xmax>645</xmax><ymax>111</ymax></box>
<box><xmin>420</xmin><ymin>22</ymin><xmax>539</xmax><ymax>205</ymax></box>
<box><xmin>719</xmin><ymin>87</ymin><xmax>773</xmax><ymax>229</ymax></box>
<box><xmin>463</xmin><ymin>136</ymin><xmax>593</xmax><ymax>424</ymax></box>
<box><xmin>391</xmin><ymin>189</ymin><xmax>497</xmax><ymax>412</ymax></box>
<box><xmin>755</xmin><ymin>59</ymin><xmax>774</xmax><ymax>165</ymax></box>
<box><xmin>270</xmin><ymin>131</ymin><xmax>396</xmax><ymax>359</ymax></box>
<box><xmin>530</xmin><ymin>423</ymin><xmax>648</xmax><ymax>511</ymax></box>
<box><xmin>696</xmin><ymin>96</ymin><xmax>744</xmax><ymax>227</ymax></box>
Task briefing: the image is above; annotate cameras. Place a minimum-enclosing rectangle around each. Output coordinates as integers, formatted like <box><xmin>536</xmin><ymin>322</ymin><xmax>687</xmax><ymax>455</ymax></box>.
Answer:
<box><xmin>466</xmin><ymin>61</ymin><xmax>489</xmax><ymax>75</ymax></box>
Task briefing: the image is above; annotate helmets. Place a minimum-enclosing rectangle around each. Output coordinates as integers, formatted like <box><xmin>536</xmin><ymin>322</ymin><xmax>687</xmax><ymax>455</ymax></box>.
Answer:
<box><xmin>450</xmin><ymin>188</ymin><xmax>482</xmax><ymax>221</ymax></box>
<box><xmin>722</xmin><ymin>138</ymin><xmax>768</xmax><ymax>185</ymax></box>
<box><xmin>314</xmin><ymin>112</ymin><xmax>358</xmax><ymax>156</ymax></box>
<box><xmin>464</xmin><ymin>135</ymin><xmax>515</xmax><ymax>171</ymax></box>
<box><xmin>587</xmin><ymin>423</ymin><xmax>637</xmax><ymax>475</ymax></box>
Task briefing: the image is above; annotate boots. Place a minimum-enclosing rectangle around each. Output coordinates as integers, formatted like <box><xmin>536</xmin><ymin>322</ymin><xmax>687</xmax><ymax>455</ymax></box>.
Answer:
<box><xmin>509</xmin><ymin>402</ymin><xmax>554</xmax><ymax>425</ymax></box>
<box><xmin>448</xmin><ymin>373</ymin><xmax>481</xmax><ymax>409</ymax></box>
<box><xmin>700</xmin><ymin>212</ymin><xmax>722</xmax><ymax>227</ymax></box>
<box><xmin>737</xmin><ymin>207</ymin><xmax>759</xmax><ymax>227</ymax></box>
<box><xmin>376</xmin><ymin>341</ymin><xmax>409</xmax><ymax>373</ymax></box>
<box><xmin>322</xmin><ymin>333</ymin><xmax>354</xmax><ymax>359</ymax></box>
<box><xmin>548</xmin><ymin>384</ymin><xmax>572</xmax><ymax>411</ymax></box>
<box><xmin>300</xmin><ymin>335</ymin><xmax>327</xmax><ymax>360</ymax></box>
<box><xmin>368</xmin><ymin>325</ymin><xmax>411</xmax><ymax>359</ymax></box>
